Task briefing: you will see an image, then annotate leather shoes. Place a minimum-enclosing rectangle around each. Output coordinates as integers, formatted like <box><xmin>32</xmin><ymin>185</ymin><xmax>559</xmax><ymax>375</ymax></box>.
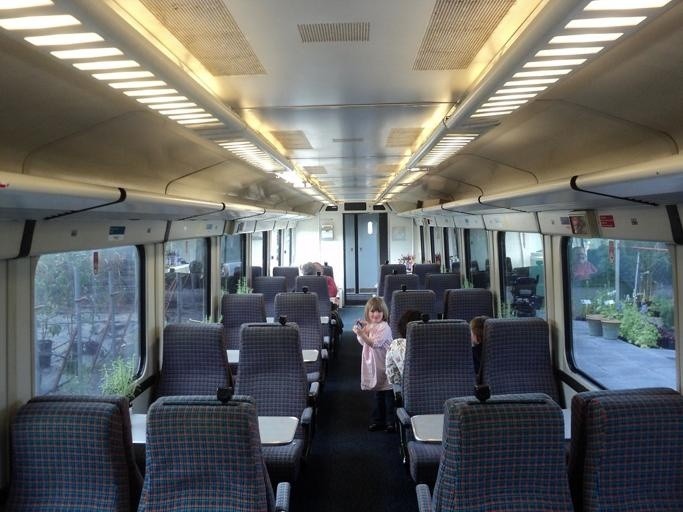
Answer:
<box><xmin>370</xmin><ymin>424</ymin><xmax>378</xmax><ymax>430</ymax></box>
<box><xmin>385</xmin><ymin>426</ymin><xmax>394</xmax><ymax>433</ymax></box>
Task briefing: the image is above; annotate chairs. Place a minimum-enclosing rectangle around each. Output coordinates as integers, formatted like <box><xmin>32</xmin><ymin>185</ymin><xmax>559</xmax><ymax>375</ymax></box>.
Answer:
<box><xmin>480</xmin><ymin>317</ymin><xmax>561</xmax><ymax>406</ymax></box>
<box><xmin>235</xmin><ymin>326</ymin><xmax>313</xmax><ymax>488</ymax></box>
<box><xmin>390</xmin><ymin>317</ymin><xmax>465</xmax><ymax>399</ymax></box>
<box><xmin>412</xmin><ymin>263</ymin><xmax>442</xmax><ymax>292</ymax></box>
<box><xmin>566</xmin><ymin>384</ymin><xmax>680</xmax><ymax>496</ymax></box>
<box><xmin>272</xmin><ymin>267</ymin><xmax>299</xmax><ymax>292</ymax></box>
<box><xmin>425</xmin><ymin>274</ymin><xmax>460</xmax><ymax>316</ymax></box>
<box><xmin>445</xmin><ymin>290</ymin><xmax>495</xmax><ymax>320</ymax></box>
<box><xmin>384</xmin><ymin>275</ymin><xmax>419</xmax><ymax>310</ymax></box>
<box><xmin>294</xmin><ymin>275</ymin><xmax>337</xmax><ymax>366</ymax></box>
<box><xmin>26</xmin><ymin>396</ymin><xmax>143</xmax><ymax>496</ymax></box>
<box><xmin>390</xmin><ymin>292</ymin><xmax>436</xmax><ymax>339</ymax></box>
<box><xmin>138</xmin><ymin>404</ymin><xmax>264</xmax><ymax>512</ymax></box>
<box><xmin>414</xmin><ymin>394</ymin><xmax>573</xmax><ymax>512</ymax></box>
<box><xmin>375</xmin><ymin>265</ymin><xmax>406</xmax><ymax>297</ymax></box>
<box><xmin>398</xmin><ymin>324</ymin><xmax>475</xmax><ymax>482</ymax></box>
<box><xmin>271</xmin><ymin>292</ymin><xmax>323</xmax><ymax>437</ymax></box>
<box><xmin>13</xmin><ymin>404</ymin><xmax>129</xmax><ymax>511</ymax></box>
<box><xmin>319</xmin><ymin>267</ymin><xmax>336</xmax><ymax>320</ymax></box>
<box><xmin>156</xmin><ymin>397</ymin><xmax>290</xmax><ymax>510</ymax></box>
<box><xmin>253</xmin><ymin>277</ymin><xmax>286</xmax><ymax>318</ymax></box>
<box><xmin>220</xmin><ymin>295</ymin><xmax>267</xmax><ymax>385</ymax></box>
<box><xmin>149</xmin><ymin>323</ymin><xmax>229</xmax><ymax>415</ymax></box>
<box><xmin>583</xmin><ymin>398</ymin><xmax>683</xmax><ymax>512</ymax></box>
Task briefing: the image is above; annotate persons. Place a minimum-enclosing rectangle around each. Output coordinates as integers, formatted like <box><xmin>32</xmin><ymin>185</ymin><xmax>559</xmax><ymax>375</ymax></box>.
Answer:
<box><xmin>352</xmin><ymin>297</ymin><xmax>396</xmax><ymax>434</ymax></box>
<box><xmin>300</xmin><ymin>262</ymin><xmax>316</xmax><ymax>275</ymax></box>
<box><xmin>469</xmin><ymin>316</ymin><xmax>492</xmax><ymax>373</ymax></box>
<box><xmin>314</xmin><ymin>262</ymin><xmax>338</xmax><ymax>297</ymax></box>
<box><xmin>405</xmin><ymin>256</ymin><xmax>415</xmax><ymax>270</ymax></box>
<box><xmin>384</xmin><ymin>311</ymin><xmax>425</xmax><ymax>401</ymax></box>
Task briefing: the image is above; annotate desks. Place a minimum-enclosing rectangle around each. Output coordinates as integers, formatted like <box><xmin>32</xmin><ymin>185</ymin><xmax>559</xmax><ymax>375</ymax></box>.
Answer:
<box><xmin>127</xmin><ymin>413</ymin><xmax>299</xmax><ymax>446</ymax></box>
<box><xmin>411</xmin><ymin>408</ymin><xmax>589</xmax><ymax>443</ymax></box>
<box><xmin>222</xmin><ymin>349</ymin><xmax>319</xmax><ymax>364</ymax></box>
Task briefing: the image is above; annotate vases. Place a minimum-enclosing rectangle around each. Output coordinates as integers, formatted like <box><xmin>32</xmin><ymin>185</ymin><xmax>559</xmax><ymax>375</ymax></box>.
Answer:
<box><xmin>601</xmin><ymin>320</ymin><xmax>622</xmax><ymax>340</ymax></box>
<box><xmin>585</xmin><ymin>314</ymin><xmax>604</xmax><ymax>335</ymax></box>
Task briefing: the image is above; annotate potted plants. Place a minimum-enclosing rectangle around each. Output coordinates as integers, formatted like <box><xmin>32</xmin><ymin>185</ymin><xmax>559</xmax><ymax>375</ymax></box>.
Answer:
<box><xmin>99</xmin><ymin>354</ymin><xmax>139</xmax><ymax>416</ymax></box>
